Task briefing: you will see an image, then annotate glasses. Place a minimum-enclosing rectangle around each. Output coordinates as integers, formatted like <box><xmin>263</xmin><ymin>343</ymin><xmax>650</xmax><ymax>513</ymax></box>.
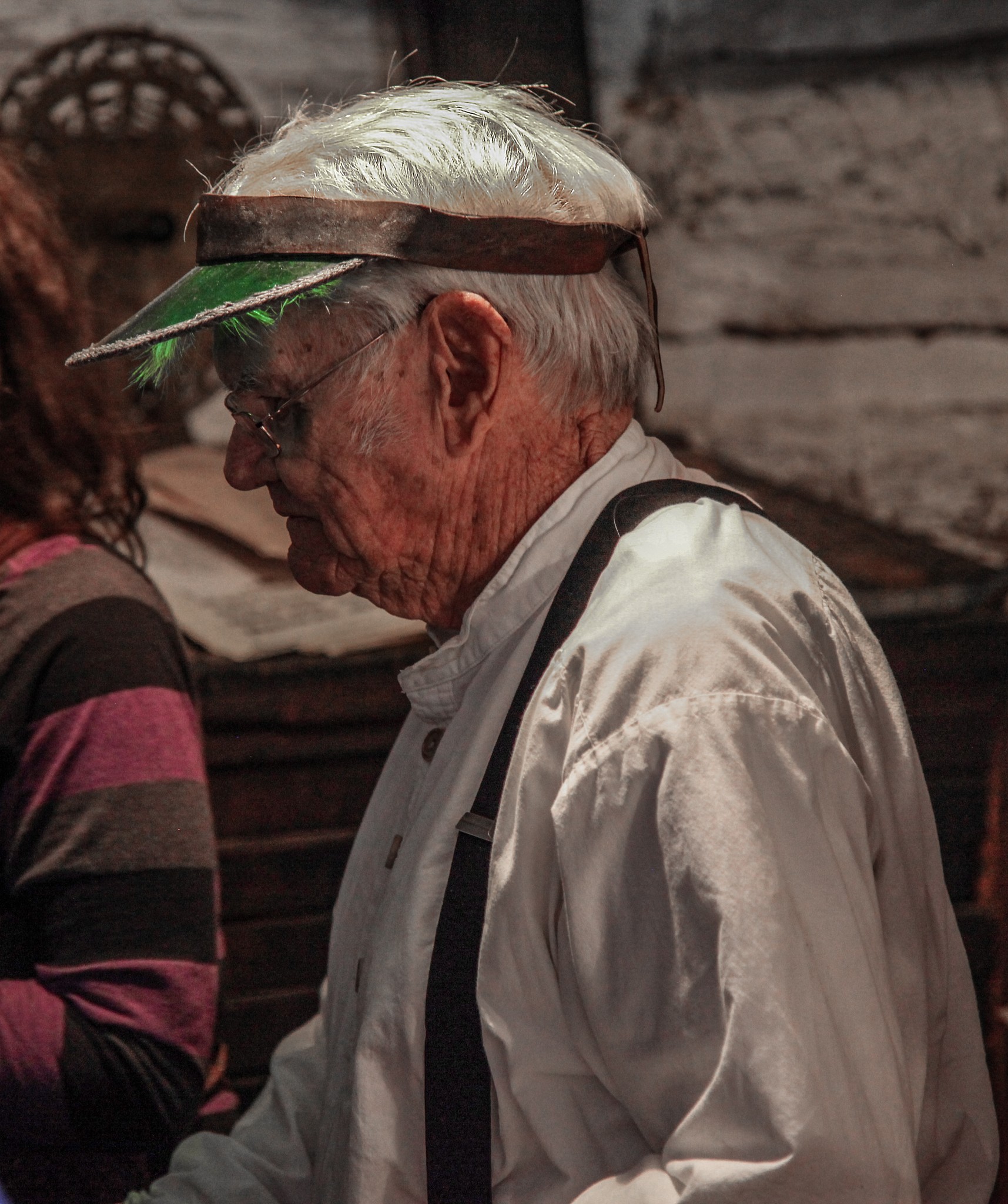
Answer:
<box><xmin>226</xmin><ymin>296</ymin><xmax>426</xmax><ymax>462</ymax></box>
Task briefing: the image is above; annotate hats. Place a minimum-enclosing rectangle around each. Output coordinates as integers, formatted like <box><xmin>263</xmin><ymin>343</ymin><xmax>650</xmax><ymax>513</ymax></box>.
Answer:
<box><xmin>63</xmin><ymin>191</ymin><xmax>652</xmax><ymax>368</ymax></box>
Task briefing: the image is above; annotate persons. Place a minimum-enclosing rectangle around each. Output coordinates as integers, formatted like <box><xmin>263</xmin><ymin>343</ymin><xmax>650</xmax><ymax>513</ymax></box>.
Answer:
<box><xmin>0</xmin><ymin>153</ymin><xmax>248</xmax><ymax>1204</ymax></box>
<box><xmin>58</xmin><ymin>66</ymin><xmax>1002</xmax><ymax>1202</ymax></box>
